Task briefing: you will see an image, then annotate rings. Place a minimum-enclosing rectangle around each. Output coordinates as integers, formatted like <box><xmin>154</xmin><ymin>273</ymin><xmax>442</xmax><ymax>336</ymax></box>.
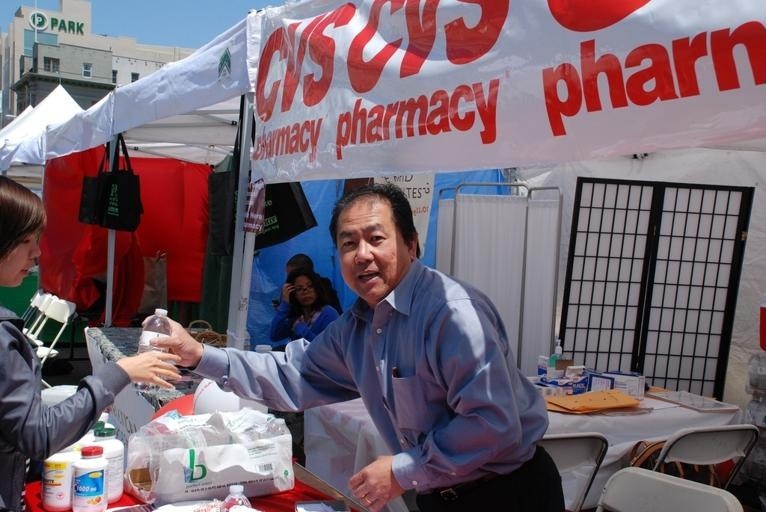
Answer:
<box><xmin>364</xmin><ymin>496</ymin><xmax>373</xmax><ymax>505</ymax></box>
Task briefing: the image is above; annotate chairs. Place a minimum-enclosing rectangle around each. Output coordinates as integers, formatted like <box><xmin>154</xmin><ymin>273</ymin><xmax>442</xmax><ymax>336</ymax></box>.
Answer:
<box><xmin>538</xmin><ymin>432</ymin><xmax>608</xmax><ymax>512</ymax></box>
<box><xmin>19</xmin><ymin>289</ymin><xmax>74</xmax><ymax>389</ymax></box>
<box><xmin>653</xmin><ymin>424</ymin><xmax>760</xmax><ymax>490</ymax></box>
<box><xmin>594</xmin><ymin>467</ymin><xmax>744</xmax><ymax>512</ymax></box>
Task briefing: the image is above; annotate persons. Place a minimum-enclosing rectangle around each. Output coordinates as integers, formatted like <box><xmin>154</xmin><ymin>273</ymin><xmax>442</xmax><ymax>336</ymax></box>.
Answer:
<box><xmin>0</xmin><ymin>173</ymin><xmax>182</xmax><ymax>512</ymax></box>
<box><xmin>139</xmin><ymin>184</ymin><xmax>566</xmax><ymax>512</ymax></box>
<box><xmin>270</xmin><ymin>253</ymin><xmax>343</xmax><ymax>349</ymax></box>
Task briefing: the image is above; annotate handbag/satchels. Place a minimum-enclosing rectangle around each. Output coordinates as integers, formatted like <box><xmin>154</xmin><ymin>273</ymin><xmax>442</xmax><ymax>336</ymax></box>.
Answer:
<box><xmin>207</xmin><ymin>154</ymin><xmax>317</xmax><ymax>256</ymax></box>
<box><xmin>79</xmin><ymin>169</ymin><xmax>143</xmax><ymax>232</ymax></box>
<box><xmin>642</xmin><ymin>443</ymin><xmax>709</xmax><ymax>482</ymax></box>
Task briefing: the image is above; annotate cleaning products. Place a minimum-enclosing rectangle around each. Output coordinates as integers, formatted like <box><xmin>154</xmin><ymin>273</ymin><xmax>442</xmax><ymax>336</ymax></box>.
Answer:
<box><xmin>45</xmin><ymin>426</ymin><xmax>126</xmax><ymax>511</ymax></box>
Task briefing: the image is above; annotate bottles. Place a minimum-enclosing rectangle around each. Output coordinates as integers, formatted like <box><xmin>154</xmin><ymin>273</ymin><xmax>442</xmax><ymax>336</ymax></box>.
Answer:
<box><xmin>220</xmin><ymin>484</ymin><xmax>254</xmax><ymax>512</ymax></box>
<box><xmin>40</xmin><ymin>419</ymin><xmax>125</xmax><ymax>512</ymax></box>
<box><xmin>131</xmin><ymin>307</ymin><xmax>174</xmax><ymax>397</ymax></box>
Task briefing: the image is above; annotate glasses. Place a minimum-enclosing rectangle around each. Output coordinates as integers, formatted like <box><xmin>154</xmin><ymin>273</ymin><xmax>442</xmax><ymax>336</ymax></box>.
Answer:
<box><xmin>294</xmin><ymin>285</ymin><xmax>314</xmax><ymax>295</ymax></box>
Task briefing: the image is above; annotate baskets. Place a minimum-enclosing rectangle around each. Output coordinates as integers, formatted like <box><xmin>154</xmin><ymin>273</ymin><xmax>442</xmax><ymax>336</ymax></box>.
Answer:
<box><xmin>187</xmin><ymin>320</ymin><xmax>227</xmax><ymax>348</ymax></box>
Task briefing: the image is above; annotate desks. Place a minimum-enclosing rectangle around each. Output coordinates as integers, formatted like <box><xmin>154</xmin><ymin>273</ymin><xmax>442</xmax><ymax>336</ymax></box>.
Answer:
<box><xmin>83</xmin><ymin>324</ymin><xmax>220</xmax><ymax>434</ymax></box>
<box><xmin>23</xmin><ymin>463</ymin><xmax>360</xmax><ymax>511</ymax></box>
<box><xmin>303</xmin><ymin>375</ymin><xmax>744</xmax><ymax>511</ymax></box>
